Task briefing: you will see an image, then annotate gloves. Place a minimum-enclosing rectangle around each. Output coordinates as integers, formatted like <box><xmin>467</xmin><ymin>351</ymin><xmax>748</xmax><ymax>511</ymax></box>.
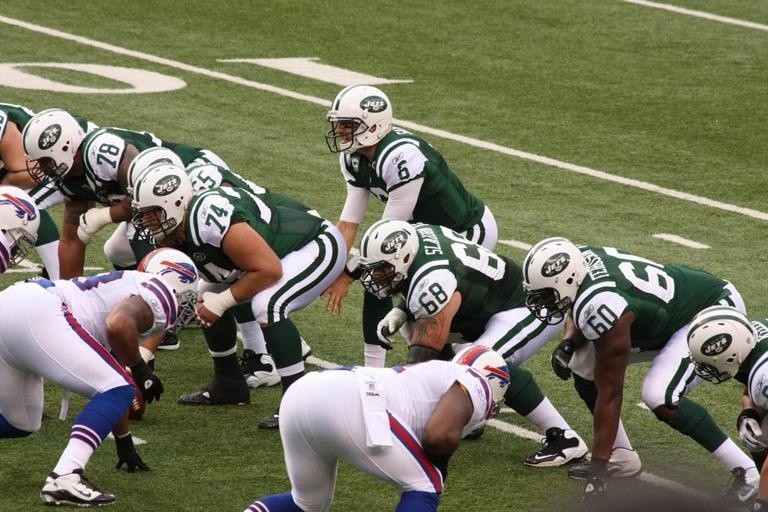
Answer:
<box><xmin>133</xmin><ymin>359</ymin><xmax>163</xmax><ymax>404</ymax></box>
<box><xmin>578</xmin><ymin>474</ymin><xmax>610</xmax><ymax>511</ymax></box>
<box><xmin>737</xmin><ymin>416</ymin><xmax>768</xmax><ymax>454</ymax></box>
<box><xmin>77</xmin><ymin>207</ymin><xmax>102</xmax><ymax>244</ymax></box>
<box><xmin>376</xmin><ymin>302</ymin><xmax>411</xmax><ymax>350</ymax></box>
<box><xmin>112</xmin><ymin>431</ymin><xmax>148</xmax><ymax>472</ymax></box>
<box><xmin>550</xmin><ymin>337</ymin><xmax>576</xmax><ymax>382</ymax></box>
<box><xmin>194</xmin><ymin>291</ymin><xmax>228</xmax><ymax>329</ymax></box>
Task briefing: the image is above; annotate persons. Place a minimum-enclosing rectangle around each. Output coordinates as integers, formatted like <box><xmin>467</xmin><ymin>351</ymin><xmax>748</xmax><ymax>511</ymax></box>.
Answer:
<box><xmin>685</xmin><ymin>304</ymin><xmax>768</xmax><ymax>511</ymax></box>
<box><xmin>315</xmin><ymin>81</ymin><xmax>503</xmax><ymax>370</ymax></box>
<box><xmin>240</xmin><ymin>341</ymin><xmax>514</xmax><ymax>512</ymax></box>
<box><xmin>519</xmin><ymin>234</ymin><xmax>760</xmax><ymax>512</ymax></box>
<box><xmin>0</xmin><ymin>102</ymin><xmax>346</xmax><ymax>507</ymax></box>
<box><xmin>355</xmin><ymin>217</ymin><xmax>593</xmax><ymax>468</ymax></box>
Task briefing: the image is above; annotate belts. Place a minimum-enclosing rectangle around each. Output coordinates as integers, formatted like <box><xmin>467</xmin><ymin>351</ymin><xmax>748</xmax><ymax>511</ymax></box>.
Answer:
<box><xmin>38</xmin><ymin>277</ymin><xmax>55</xmax><ymax>289</ymax></box>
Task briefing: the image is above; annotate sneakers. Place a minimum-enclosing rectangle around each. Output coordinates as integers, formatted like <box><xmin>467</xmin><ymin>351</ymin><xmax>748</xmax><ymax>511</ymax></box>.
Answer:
<box><xmin>717</xmin><ymin>465</ymin><xmax>761</xmax><ymax>511</ymax></box>
<box><xmin>41</xmin><ymin>468</ymin><xmax>118</xmax><ymax>507</ymax></box>
<box><xmin>157</xmin><ymin>333</ymin><xmax>311</xmax><ymax>431</ymax></box>
<box><xmin>524</xmin><ymin>428</ymin><xmax>588</xmax><ymax>468</ymax></box>
<box><xmin>569</xmin><ymin>446</ymin><xmax>642</xmax><ymax>481</ymax></box>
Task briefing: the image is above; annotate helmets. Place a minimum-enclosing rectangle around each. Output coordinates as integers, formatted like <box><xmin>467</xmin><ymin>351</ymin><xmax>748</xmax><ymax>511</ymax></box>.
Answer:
<box><xmin>23</xmin><ymin>107</ymin><xmax>85</xmax><ymax>193</ymax></box>
<box><xmin>139</xmin><ymin>246</ymin><xmax>201</xmax><ymax>332</ymax></box>
<box><xmin>357</xmin><ymin>218</ymin><xmax>421</xmax><ymax>302</ymax></box>
<box><xmin>324</xmin><ymin>85</ymin><xmax>393</xmax><ymax>155</ymax></box>
<box><xmin>450</xmin><ymin>343</ymin><xmax>511</xmax><ymax>419</ymax></box>
<box><xmin>125</xmin><ymin>146</ymin><xmax>193</xmax><ymax>247</ymax></box>
<box><xmin>686</xmin><ymin>305</ymin><xmax>759</xmax><ymax>386</ymax></box>
<box><xmin>0</xmin><ymin>186</ymin><xmax>42</xmax><ymax>276</ymax></box>
<box><xmin>520</xmin><ymin>238</ymin><xmax>589</xmax><ymax>326</ymax></box>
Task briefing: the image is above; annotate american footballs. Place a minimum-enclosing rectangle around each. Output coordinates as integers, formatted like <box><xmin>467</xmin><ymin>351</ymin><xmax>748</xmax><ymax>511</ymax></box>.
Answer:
<box><xmin>122</xmin><ymin>370</ymin><xmax>147</xmax><ymax>420</ymax></box>
